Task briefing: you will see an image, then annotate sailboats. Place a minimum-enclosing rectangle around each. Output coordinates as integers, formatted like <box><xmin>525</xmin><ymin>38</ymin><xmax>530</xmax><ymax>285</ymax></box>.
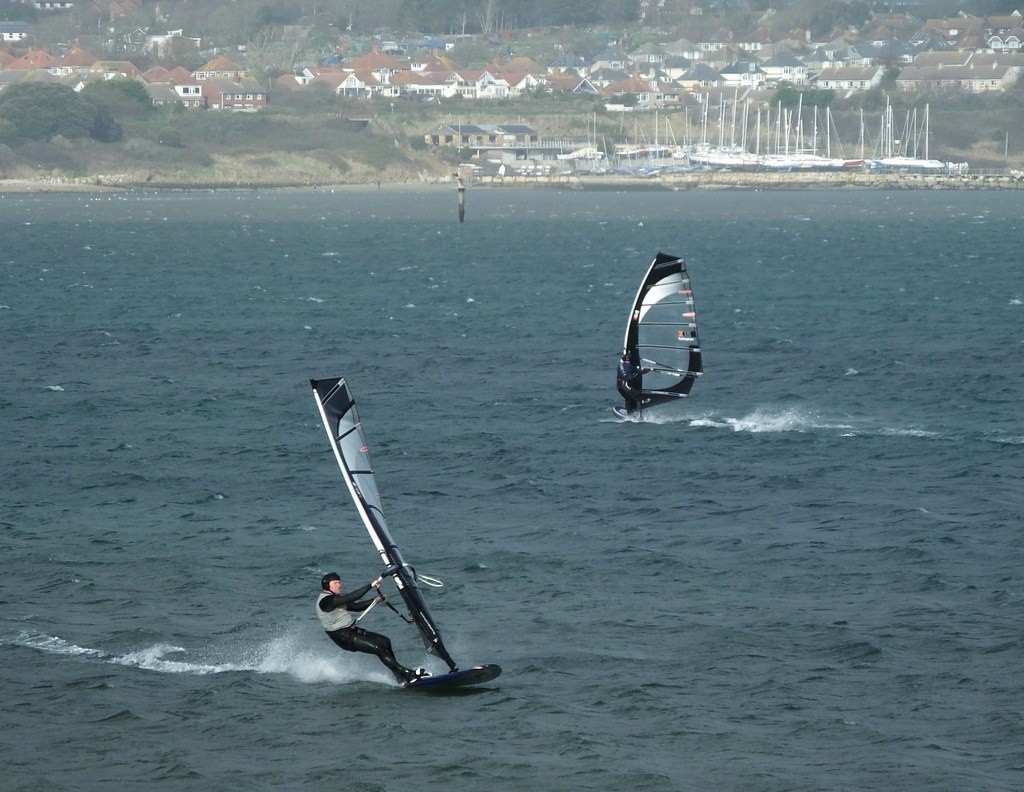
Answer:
<box><xmin>613</xmin><ymin>253</ymin><xmax>702</xmax><ymax>426</ymax></box>
<box><xmin>558</xmin><ymin>87</ymin><xmax>945</xmax><ymax>176</ymax></box>
<box><xmin>307</xmin><ymin>378</ymin><xmax>502</xmax><ymax>696</ymax></box>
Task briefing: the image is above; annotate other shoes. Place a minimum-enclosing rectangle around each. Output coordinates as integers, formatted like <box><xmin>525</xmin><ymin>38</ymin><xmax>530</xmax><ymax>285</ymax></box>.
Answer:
<box><xmin>413</xmin><ymin>667</ymin><xmax>434</xmax><ymax>678</ymax></box>
<box><xmin>395</xmin><ymin>666</ymin><xmax>421</xmax><ymax>685</ymax></box>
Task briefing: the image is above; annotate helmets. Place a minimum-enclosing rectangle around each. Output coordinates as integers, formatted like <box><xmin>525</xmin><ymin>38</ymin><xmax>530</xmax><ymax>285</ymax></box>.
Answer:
<box><xmin>621</xmin><ymin>353</ymin><xmax>633</xmax><ymax>361</ymax></box>
<box><xmin>321</xmin><ymin>573</ymin><xmax>341</xmax><ymax>591</ymax></box>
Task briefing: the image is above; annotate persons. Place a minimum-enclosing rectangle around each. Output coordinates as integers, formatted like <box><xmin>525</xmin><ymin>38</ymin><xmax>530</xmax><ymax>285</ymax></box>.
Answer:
<box><xmin>616</xmin><ymin>352</ymin><xmax>654</xmax><ymax>420</ymax></box>
<box><xmin>315</xmin><ymin>572</ymin><xmax>432</xmax><ymax>689</ymax></box>
